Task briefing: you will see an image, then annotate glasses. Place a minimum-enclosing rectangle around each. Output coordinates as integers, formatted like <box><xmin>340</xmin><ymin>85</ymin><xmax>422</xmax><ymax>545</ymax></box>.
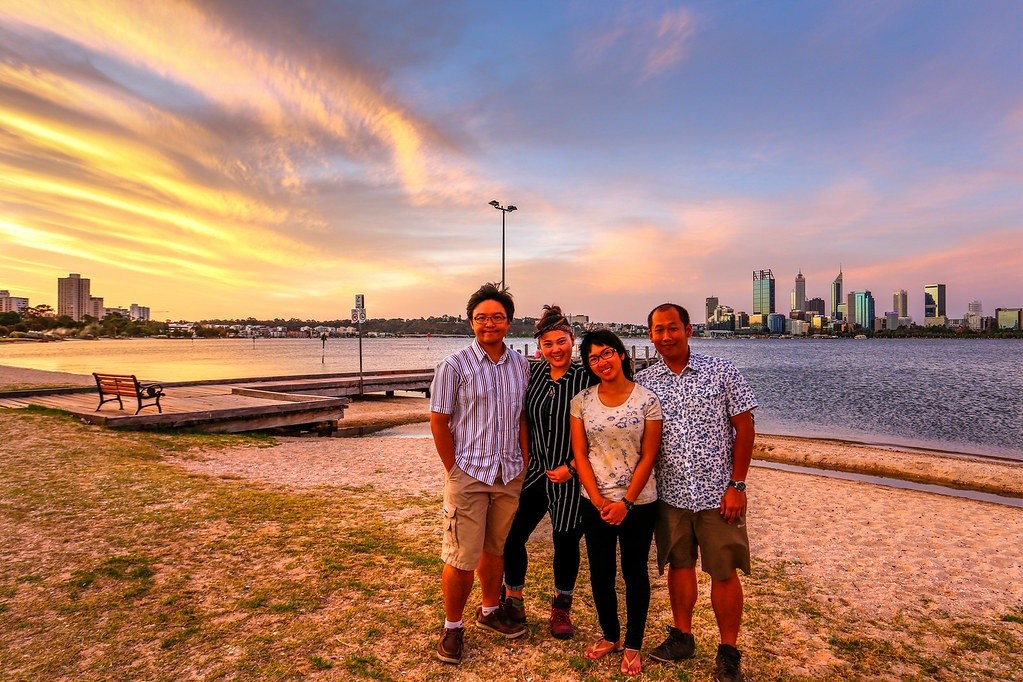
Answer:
<box><xmin>588</xmin><ymin>348</ymin><xmax>616</xmax><ymax>367</ymax></box>
<box><xmin>471</xmin><ymin>314</ymin><xmax>507</xmax><ymax>324</ymax></box>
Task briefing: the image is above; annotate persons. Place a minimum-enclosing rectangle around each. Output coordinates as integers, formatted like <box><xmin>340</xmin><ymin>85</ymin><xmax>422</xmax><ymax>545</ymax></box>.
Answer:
<box><xmin>568</xmin><ymin>328</ymin><xmax>663</xmax><ymax>674</ymax></box>
<box><xmin>428</xmin><ymin>279</ymin><xmax>532</xmax><ymax>663</ymax></box>
<box><xmin>625</xmin><ymin>303</ymin><xmax>758</xmax><ymax>682</ymax></box>
<box><xmin>486</xmin><ymin>304</ymin><xmax>595</xmax><ymax>638</ymax></box>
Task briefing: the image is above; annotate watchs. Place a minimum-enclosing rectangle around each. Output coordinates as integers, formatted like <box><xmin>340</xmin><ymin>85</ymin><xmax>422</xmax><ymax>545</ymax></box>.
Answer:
<box><xmin>621</xmin><ymin>497</ymin><xmax>634</xmax><ymax>511</ymax></box>
<box><xmin>728</xmin><ymin>481</ymin><xmax>747</xmax><ymax>492</ymax></box>
<box><xmin>566</xmin><ymin>463</ymin><xmax>577</xmax><ymax>477</ymax></box>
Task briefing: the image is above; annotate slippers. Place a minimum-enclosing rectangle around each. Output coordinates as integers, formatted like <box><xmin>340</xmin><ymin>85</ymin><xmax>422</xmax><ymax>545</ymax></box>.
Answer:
<box><xmin>584</xmin><ymin>640</ymin><xmax>620</xmax><ymax>659</ymax></box>
<box><xmin>622</xmin><ymin>650</ymin><xmax>642</xmax><ymax>675</ymax></box>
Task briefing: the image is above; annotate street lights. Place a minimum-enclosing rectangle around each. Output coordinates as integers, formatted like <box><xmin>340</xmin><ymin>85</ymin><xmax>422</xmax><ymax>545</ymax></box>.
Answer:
<box><xmin>488</xmin><ymin>200</ymin><xmax>517</xmax><ymax>293</ymax></box>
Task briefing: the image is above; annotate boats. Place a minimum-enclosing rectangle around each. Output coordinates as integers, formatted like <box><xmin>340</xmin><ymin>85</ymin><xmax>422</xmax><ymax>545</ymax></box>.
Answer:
<box><xmin>768</xmin><ymin>335</ymin><xmax>839</xmax><ymax>340</ymax></box>
<box><xmin>715</xmin><ymin>335</ymin><xmax>757</xmax><ymax>340</ymax></box>
<box><xmin>854</xmin><ymin>335</ymin><xmax>868</xmax><ymax>340</ymax></box>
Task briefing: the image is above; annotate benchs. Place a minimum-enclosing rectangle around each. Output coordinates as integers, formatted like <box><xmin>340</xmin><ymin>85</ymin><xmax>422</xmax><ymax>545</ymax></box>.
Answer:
<box><xmin>92</xmin><ymin>372</ymin><xmax>167</xmax><ymax>415</ymax></box>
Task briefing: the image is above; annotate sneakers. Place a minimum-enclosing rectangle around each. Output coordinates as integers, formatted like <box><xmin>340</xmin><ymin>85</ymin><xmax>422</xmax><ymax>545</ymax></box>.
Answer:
<box><xmin>476</xmin><ymin>585</ymin><xmax>526</xmax><ymax>623</ymax></box>
<box><xmin>436</xmin><ymin>625</ymin><xmax>466</xmax><ymax>663</ymax></box>
<box><xmin>648</xmin><ymin>625</ymin><xmax>695</xmax><ymax>662</ymax></box>
<box><xmin>549</xmin><ymin>606</ymin><xmax>574</xmax><ymax>640</ymax></box>
<box><xmin>475</xmin><ymin>605</ymin><xmax>527</xmax><ymax>638</ymax></box>
<box><xmin>715</xmin><ymin>643</ymin><xmax>743</xmax><ymax>682</ymax></box>
